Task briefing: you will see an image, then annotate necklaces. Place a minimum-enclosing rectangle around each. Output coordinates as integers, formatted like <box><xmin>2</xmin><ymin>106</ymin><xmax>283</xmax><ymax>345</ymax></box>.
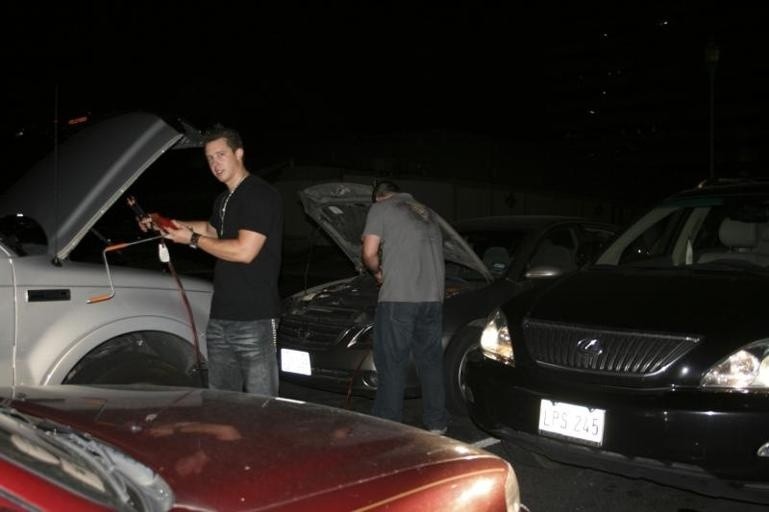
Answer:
<box><xmin>218</xmin><ymin>172</ymin><xmax>250</xmax><ymax>236</ymax></box>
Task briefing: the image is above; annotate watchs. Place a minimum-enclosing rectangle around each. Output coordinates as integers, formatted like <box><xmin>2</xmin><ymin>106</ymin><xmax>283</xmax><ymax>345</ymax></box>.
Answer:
<box><xmin>189</xmin><ymin>233</ymin><xmax>200</xmax><ymax>249</ymax></box>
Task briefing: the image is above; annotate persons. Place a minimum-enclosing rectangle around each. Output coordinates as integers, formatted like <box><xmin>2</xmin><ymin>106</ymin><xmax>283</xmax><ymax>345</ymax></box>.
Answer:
<box><xmin>135</xmin><ymin>128</ymin><xmax>282</xmax><ymax>397</ymax></box>
<box><xmin>360</xmin><ymin>181</ymin><xmax>450</xmax><ymax>435</ymax></box>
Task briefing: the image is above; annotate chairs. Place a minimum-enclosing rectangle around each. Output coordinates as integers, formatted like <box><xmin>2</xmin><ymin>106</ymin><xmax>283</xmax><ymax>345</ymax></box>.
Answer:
<box><xmin>696</xmin><ymin>218</ymin><xmax>768</xmax><ymax>267</ymax></box>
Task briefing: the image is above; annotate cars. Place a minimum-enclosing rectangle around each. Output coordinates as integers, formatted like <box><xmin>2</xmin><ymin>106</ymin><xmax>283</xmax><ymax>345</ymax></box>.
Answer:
<box><xmin>0</xmin><ymin>109</ymin><xmax>216</xmax><ymax>387</ymax></box>
<box><xmin>274</xmin><ymin>178</ymin><xmax>625</xmax><ymax>418</ymax></box>
<box><xmin>463</xmin><ymin>181</ymin><xmax>769</xmax><ymax>507</ymax></box>
<box><xmin>0</xmin><ymin>380</ymin><xmax>520</xmax><ymax>512</ymax></box>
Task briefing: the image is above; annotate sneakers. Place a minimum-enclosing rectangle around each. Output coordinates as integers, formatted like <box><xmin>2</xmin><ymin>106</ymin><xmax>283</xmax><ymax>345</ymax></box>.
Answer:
<box><xmin>430</xmin><ymin>426</ymin><xmax>448</xmax><ymax>434</ymax></box>
